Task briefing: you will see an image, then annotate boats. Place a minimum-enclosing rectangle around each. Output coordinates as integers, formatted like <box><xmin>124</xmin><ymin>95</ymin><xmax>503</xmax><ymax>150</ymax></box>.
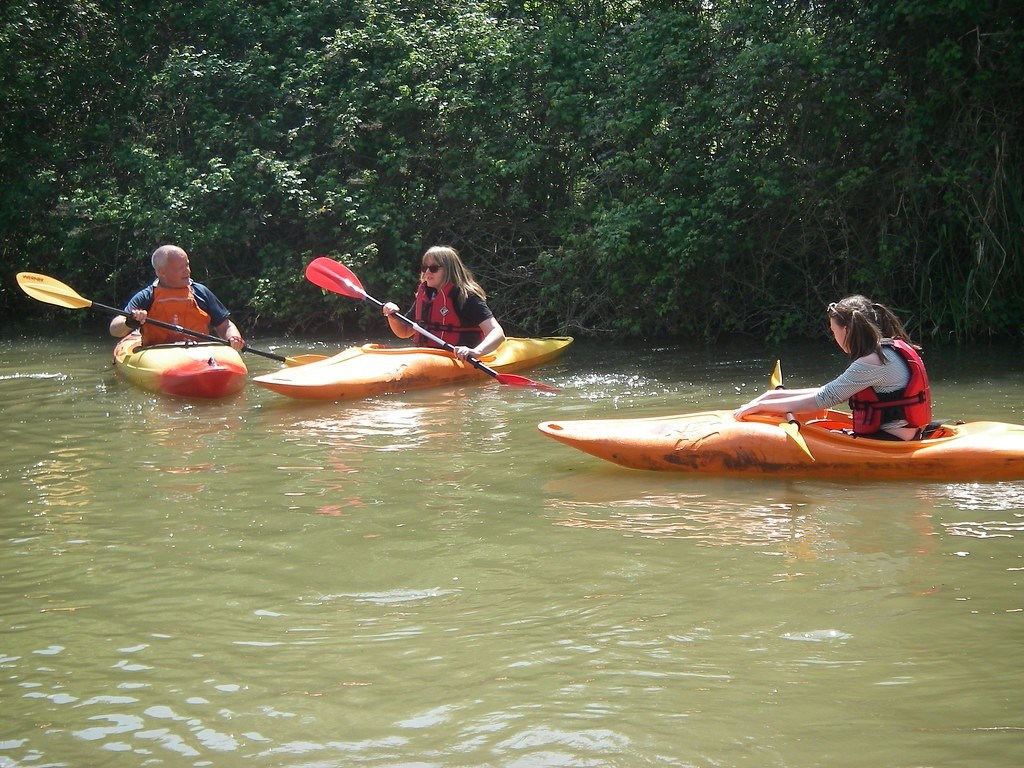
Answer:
<box><xmin>537</xmin><ymin>406</ymin><xmax>1024</xmax><ymax>488</ymax></box>
<box><xmin>112</xmin><ymin>327</ymin><xmax>249</xmax><ymax>399</ymax></box>
<box><xmin>252</xmin><ymin>334</ymin><xmax>574</xmax><ymax>401</ymax></box>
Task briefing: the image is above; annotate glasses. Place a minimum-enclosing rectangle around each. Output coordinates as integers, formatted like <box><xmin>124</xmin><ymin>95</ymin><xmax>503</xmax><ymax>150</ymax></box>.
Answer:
<box><xmin>421</xmin><ymin>264</ymin><xmax>443</xmax><ymax>273</ymax></box>
<box><xmin>828</xmin><ymin>302</ymin><xmax>846</xmax><ymax>322</ymax></box>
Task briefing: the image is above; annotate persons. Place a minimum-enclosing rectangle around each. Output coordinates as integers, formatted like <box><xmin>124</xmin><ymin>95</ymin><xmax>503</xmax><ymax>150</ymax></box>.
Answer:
<box><xmin>109</xmin><ymin>245</ymin><xmax>248</xmax><ymax>354</ymax></box>
<box><xmin>380</xmin><ymin>245</ymin><xmax>506</xmax><ymax>362</ymax></box>
<box><xmin>732</xmin><ymin>295</ymin><xmax>932</xmax><ymax>440</ymax></box>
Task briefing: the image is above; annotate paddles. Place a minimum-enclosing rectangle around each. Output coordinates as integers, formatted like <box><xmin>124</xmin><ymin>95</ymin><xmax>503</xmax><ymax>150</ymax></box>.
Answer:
<box><xmin>305</xmin><ymin>254</ymin><xmax>547</xmax><ymax>386</ymax></box>
<box><xmin>15</xmin><ymin>269</ymin><xmax>328</xmax><ymax>365</ymax></box>
<box><xmin>769</xmin><ymin>359</ymin><xmax>818</xmax><ymax>461</ymax></box>
